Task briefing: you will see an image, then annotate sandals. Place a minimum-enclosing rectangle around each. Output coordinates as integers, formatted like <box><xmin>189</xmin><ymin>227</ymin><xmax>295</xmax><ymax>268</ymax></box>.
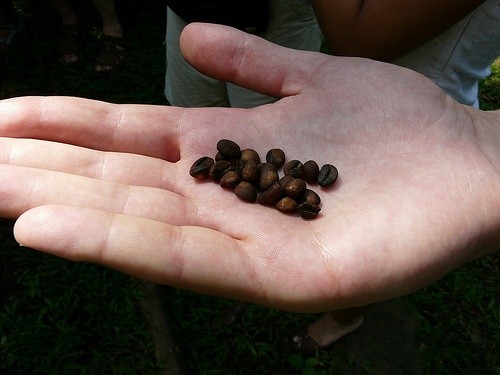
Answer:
<box><xmin>56</xmin><ymin>24</ymin><xmax>84</xmax><ymax>64</ymax></box>
<box><xmin>93</xmin><ymin>36</ymin><xmax>127</xmax><ymax>79</ymax></box>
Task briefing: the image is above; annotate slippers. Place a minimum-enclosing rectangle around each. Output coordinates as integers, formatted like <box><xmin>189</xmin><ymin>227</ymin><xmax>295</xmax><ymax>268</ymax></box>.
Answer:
<box><xmin>292</xmin><ymin>322</ymin><xmax>363</xmax><ymax>354</ymax></box>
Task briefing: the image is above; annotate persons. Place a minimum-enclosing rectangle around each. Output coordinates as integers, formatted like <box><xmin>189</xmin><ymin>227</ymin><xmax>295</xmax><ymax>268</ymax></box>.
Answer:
<box><xmin>0</xmin><ymin>0</ymin><xmax>499</xmax><ymax>375</ymax></box>
